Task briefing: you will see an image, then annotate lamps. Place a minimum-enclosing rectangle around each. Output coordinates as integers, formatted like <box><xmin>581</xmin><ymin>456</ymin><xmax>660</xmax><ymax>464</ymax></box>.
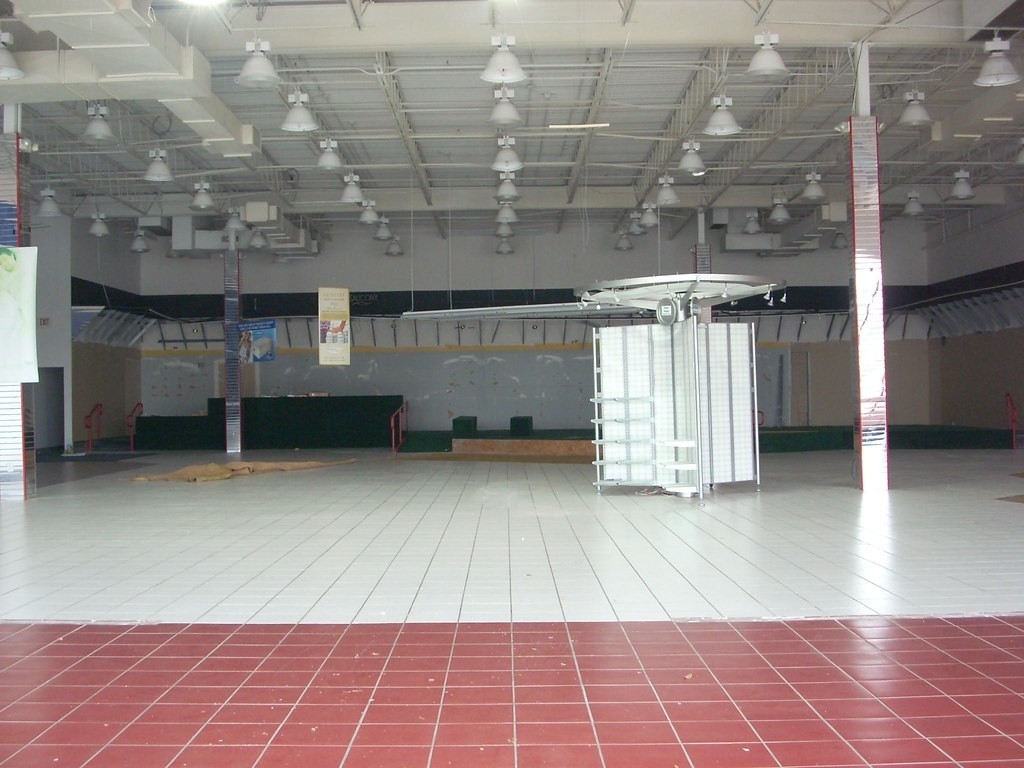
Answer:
<box><xmin>802</xmin><ymin>170</ymin><xmax>826</xmax><ymax>202</ymax></box>
<box><xmin>626</xmin><ymin>212</ymin><xmax>648</xmax><ymax>235</ymax></box>
<box><xmin>833</xmin><ymin>232</ymin><xmax>850</xmax><ymax>249</ymax></box>
<box><xmin>616</xmin><ymin>232</ymin><xmax>634</xmax><ymax>252</ymax></box>
<box><xmin>492</xmin><ymin>174</ymin><xmax>520</xmax><ymax>255</ymax></box>
<box><xmin>487</xmin><ymin>87</ymin><xmax>523</xmax><ymax>128</ymax></box>
<box><xmin>480</xmin><ymin>34</ymin><xmax>531</xmax><ymax>84</ymax></box>
<box><xmin>951</xmin><ymin>171</ymin><xmax>977</xmax><ymax>201</ymax></box>
<box><xmin>896</xmin><ymin>86</ymin><xmax>935</xmax><ymax>129</ymax></box>
<box><xmin>973</xmin><ymin>40</ymin><xmax>1023</xmax><ymax>88</ymax></box>
<box><xmin>676</xmin><ymin>139</ymin><xmax>706</xmax><ymax>176</ymax></box>
<box><xmin>744</xmin><ymin>29</ymin><xmax>791</xmax><ymax>87</ymax></box>
<box><xmin>742</xmin><ymin>210</ymin><xmax>762</xmax><ymax>234</ymax></box>
<box><xmin>768</xmin><ymin>196</ymin><xmax>792</xmax><ymax>225</ymax></box>
<box><xmin>639</xmin><ymin>203</ymin><xmax>661</xmax><ymax>228</ymax></box>
<box><xmin>701</xmin><ymin>91</ymin><xmax>742</xmax><ymax>138</ymax></box>
<box><xmin>902</xmin><ymin>189</ymin><xmax>924</xmax><ymax>217</ymax></box>
<box><xmin>655</xmin><ymin>175</ymin><xmax>681</xmax><ymax>206</ymax></box>
<box><xmin>37</xmin><ymin>41</ymin><xmax>405</xmax><ymax>257</ymax></box>
<box><xmin>491</xmin><ymin>135</ymin><xmax>524</xmax><ymax>173</ymax></box>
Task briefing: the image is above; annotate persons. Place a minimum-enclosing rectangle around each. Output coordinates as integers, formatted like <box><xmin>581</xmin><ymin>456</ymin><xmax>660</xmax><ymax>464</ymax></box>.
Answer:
<box><xmin>238</xmin><ymin>332</ymin><xmax>252</xmax><ymax>364</ymax></box>
<box><xmin>321</xmin><ymin>321</ymin><xmax>330</xmax><ymax>343</ymax></box>
<box><xmin>0</xmin><ymin>248</ymin><xmax>20</xmax><ymax>275</ymax></box>
